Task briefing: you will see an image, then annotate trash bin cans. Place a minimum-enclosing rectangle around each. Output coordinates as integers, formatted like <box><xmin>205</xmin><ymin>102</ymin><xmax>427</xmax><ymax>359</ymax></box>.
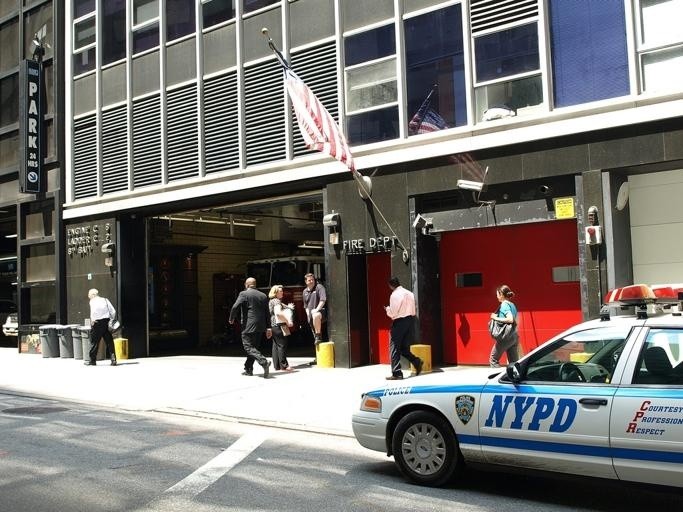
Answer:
<box><xmin>39</xmin><ymin>323</ymin><xmax>106</xmax><ymax>361</ymax></box>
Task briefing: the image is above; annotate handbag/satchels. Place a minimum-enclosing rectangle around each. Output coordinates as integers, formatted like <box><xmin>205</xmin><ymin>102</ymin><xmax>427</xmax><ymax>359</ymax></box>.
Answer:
<box><xmin>280</xmin><ymin>322</ymin><xmax>291</xmax><ymax>337</ymax></box>
<box><xmin>487</xmin><ymin>301</ymin><xmax>517</xmax><ymax>344</ymax></box>
<box><xmin>108</xmin><ymin>319</ymin><xmax>121</xmax><ymax>333</ymax></box>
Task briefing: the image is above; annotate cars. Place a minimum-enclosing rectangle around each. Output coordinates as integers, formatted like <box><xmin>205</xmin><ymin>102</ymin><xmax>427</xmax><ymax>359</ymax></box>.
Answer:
<box><xmin>352</xmin><ymin>283</ymin><xmax>683</xmax><ymax>489</ymax></box>
<box><xmin>0</xmin><ymin>299</ymin><xmax>19</xmax><ymax>343</ymax></box>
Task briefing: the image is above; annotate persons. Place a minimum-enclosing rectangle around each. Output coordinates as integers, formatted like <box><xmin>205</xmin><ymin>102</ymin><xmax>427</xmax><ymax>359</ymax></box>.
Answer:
<box><xmin>488</xmin><ymin>284</ymin><xmax>520</xmax><ymax>368</ymax></box>
<box><xmin>83</xmin><ymin>287</ymin><xmax>119</xmax><ymax>367</ymax></box>
<box><xmin>302</xmin><ymin>272</ymin><xmax>327</xmax><ymax>369</ymax></box>
<box><xmin>227</xmin><ymin>277</ymin><xmax>273</xmax><ymax>379</ymax></box>
<box><xmin>267</xmin><ymin>284</ymin><xmax>296</xmax><ymax>373</ymax></box>
<box><xmin>383</xmin><ymin>277</ymin><xmax>424</xmax><ymax>381</ymax></box>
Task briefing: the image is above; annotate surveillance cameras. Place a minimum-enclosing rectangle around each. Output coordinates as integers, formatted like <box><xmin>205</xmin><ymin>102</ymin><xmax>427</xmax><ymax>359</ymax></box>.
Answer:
<box><xmin>456</xmin><ymin>179</ymin><xmax>487</xmax><ymax>193</ymax></box>
<box><xmin>539</xmin><ymin>184</ymin><xmax>550</xmax><ymax>194</ymax></box>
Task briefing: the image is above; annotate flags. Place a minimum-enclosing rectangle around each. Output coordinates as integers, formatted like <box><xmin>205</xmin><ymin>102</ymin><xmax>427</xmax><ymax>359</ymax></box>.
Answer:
<box><xmin>269</xmin><ymin>41</ymin><xmax>357</xmax><ymax>174</ymax></box>
<box><xmin>408</xmin><ymin>83</ymin><xmax>489</xmax><ymax>190</ymax></box>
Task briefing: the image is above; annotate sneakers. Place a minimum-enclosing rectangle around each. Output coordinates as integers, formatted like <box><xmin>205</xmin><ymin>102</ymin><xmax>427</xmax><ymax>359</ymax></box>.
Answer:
<box><xmin>385</xmin><ymin>374</ymin><xmax>403</xmax><ymax>381</ymax></box>
<box><xmin>83</xmin><ymin>362</ymin><xmax>96</xmax><ymax>366</ymax></box>
<box><xmin>263</xmin><ymin>360</ymin><xmax>271</xmax><ymax>379</ymax></box>
<box><xmin>416</xmin><ymin>359</ymin><xmax>423</xmax><ymax>377</ymax></box>
<box><xmin>241</xmin><ymin>371</ymin><xmax>253</xmax><ymax>376</ymax></box>
<box><xmin>110</xmin><ymin>360</ymin><xmax>117</xmax><ymax>365</ymax></box>
<box><xmin>314</xmin><ymin>337</ymin><xmax>322</xmax><ymax>345</ymax></box>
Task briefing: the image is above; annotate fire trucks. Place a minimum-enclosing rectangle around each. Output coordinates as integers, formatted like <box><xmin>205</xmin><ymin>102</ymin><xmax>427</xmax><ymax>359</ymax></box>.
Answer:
<box><xmin>245</xmin><ymin>256</ymin><xmax>324</xmax><ymax>347</ymax></box>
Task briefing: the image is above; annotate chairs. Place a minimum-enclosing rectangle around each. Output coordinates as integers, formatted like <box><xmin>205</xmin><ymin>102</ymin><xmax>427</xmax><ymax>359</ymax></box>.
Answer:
<box><xmin>643</xmin><ymin>346</ymin><xmax>672</xmax><ymax>374</ymax></box>
